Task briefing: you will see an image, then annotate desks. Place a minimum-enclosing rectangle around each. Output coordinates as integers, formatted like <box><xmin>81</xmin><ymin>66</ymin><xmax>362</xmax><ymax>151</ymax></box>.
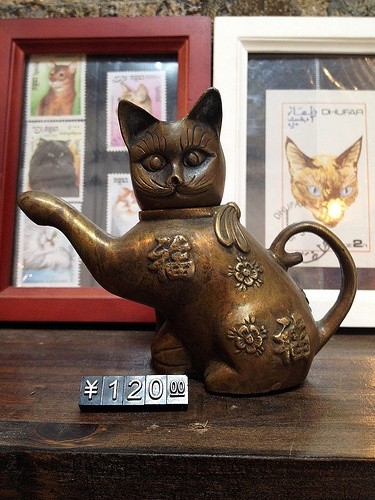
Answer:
<box><xmin>0</xmin><ymin>316</ymin><xmax>375</xmax><ymax>500</ymax></box>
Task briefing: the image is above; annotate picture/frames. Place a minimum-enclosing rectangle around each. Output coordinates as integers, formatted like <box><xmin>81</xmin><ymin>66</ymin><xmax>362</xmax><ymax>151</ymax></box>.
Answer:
<box><xmin>0</xmin><ymin>16</ymin><xmax>214</xmax><ymax>322</ymax></box>
<box><xmin>214</xmin><ymin>15</ymin><xmax>375</xmax><ymax>330</ymax></box>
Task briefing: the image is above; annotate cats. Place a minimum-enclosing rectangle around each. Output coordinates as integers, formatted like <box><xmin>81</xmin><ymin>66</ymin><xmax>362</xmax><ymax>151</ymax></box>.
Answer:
<box><xmin>28</xmin><ymin>137</ymin><xmax>80</xmax><ymax>198</ymax></box>
<box><xmin>112</xmin><ymin>187</ymin><xmax>143</xmax><ymax>237</ymax></box>
<box><xmin>15</xmin><ymin>90</ymin><xmax>357</xmax><ymax>396</ymax></box>
<box><xmin>36</xmin><ymin>57</ymin><xmax>79</xmax><ymax>116</ymax></box>
